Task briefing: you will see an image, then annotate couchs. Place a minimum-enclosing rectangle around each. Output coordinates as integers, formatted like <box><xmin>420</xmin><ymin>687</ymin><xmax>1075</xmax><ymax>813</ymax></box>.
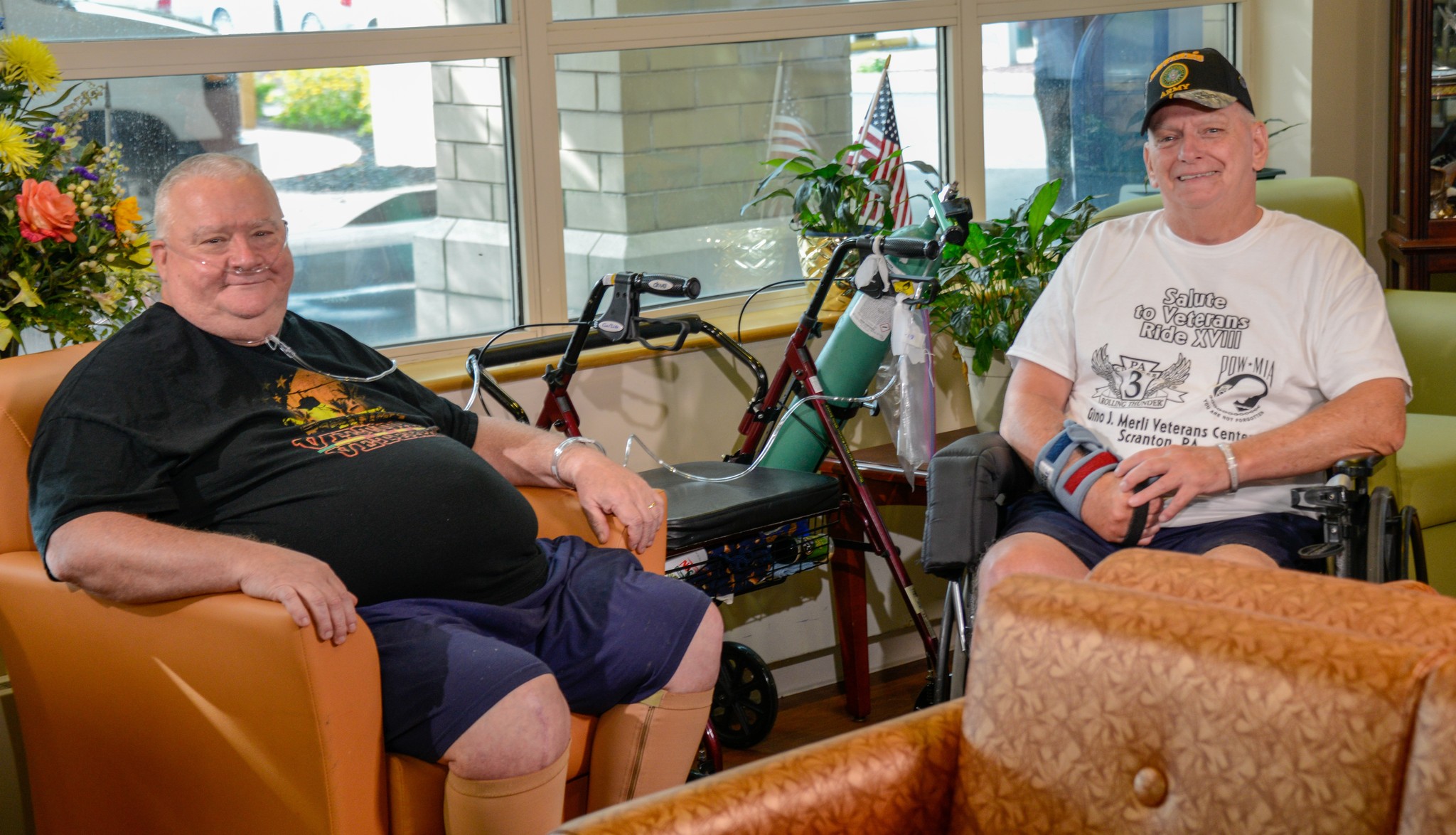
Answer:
<box><xmin>525</xmin><ymin>541</ymin><xmax>1456</xmax><ymax>835</ymax></box>
<box><xmin>1074</xmin><ymin>173</ymin><xmax>1456</xmax><ymax>587</ymax></box>
<box><xmin>0</xmin><ymin>346</ymin><xmax>681</xmax><ymax>835</ymax></box>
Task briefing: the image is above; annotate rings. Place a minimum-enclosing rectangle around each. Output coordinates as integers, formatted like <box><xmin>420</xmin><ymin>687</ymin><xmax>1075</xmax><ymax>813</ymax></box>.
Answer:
<box><xmin>648</xmin><ymin>503</ymin><xmax>656</xmax><ymax>509</ymax></box>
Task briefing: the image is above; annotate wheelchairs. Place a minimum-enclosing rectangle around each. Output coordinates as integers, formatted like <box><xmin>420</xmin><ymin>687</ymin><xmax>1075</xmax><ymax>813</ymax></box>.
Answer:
<box><xmin>461</xmin><ymin>234</ymin><xmax>956</xmax><ymax>783</ymax></box>
<box><xmin>920</xmin><ymin>432</ymin><xmax>1431</xmax><ymax>710</ymax></box>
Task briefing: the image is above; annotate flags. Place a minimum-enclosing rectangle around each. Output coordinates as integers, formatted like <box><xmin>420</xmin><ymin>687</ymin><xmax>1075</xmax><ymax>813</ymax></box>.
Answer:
<box><xmin>762</xmin><ymin>63</ymin><xmax>826</xmax><ymax>172</ymax></box>
<box><xmin>846</xmin><ymin>72</ymin><xmax>913</xmax><ymax>231</ymax></box>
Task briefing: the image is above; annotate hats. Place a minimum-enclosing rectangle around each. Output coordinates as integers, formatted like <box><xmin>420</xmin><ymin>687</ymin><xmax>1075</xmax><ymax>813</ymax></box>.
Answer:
<box><xmin>1139</xmin><ymin>48</ymin><xmax>1258</xmax><ymax>136</ymax></box>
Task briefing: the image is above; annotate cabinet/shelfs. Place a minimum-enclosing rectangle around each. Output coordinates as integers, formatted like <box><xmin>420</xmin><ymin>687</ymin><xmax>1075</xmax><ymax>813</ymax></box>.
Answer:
<box><xmin>1387</xmin><ymin>0</ymin><xmax>1456</xmax><ymax>290</ymax></box>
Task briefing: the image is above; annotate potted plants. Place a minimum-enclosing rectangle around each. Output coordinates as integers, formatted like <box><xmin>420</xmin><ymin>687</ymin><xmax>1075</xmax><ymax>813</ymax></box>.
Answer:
<box><xmin>896</xmin><ymin>178</ymin><xmax>1100</xmax><ymax>440</ymax></box>
<box><xmin>740</xmin><ymin>142</ymin><xmax>941</xmax><ymax>322</ymax></box>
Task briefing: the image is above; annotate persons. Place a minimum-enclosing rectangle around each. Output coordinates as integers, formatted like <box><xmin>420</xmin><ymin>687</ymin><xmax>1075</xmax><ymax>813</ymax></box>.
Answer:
<box><xmin>28</xmin><ymin>153</ymin><xmax>723</xmax><ymax>835</ymax></box>
<box><xmin>1031</xmin><ymin>15</ymin><xmax>1096</xmax><ymax>221</ymax></box>
<box><xmin>976</xmin><ymin>48</ymin><xmax>1415</xmax><ymax>609</ymax></box>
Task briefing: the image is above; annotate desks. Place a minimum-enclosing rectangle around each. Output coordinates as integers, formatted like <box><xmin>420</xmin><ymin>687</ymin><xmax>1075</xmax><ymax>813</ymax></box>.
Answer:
<box><xmin>815</xmin><ymin>425</ymin><xmax>982</xmax><ymax>721</ymax></box>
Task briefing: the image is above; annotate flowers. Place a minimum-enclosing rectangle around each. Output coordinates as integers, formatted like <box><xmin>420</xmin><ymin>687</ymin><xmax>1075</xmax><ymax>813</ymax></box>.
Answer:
<box><xmin>0</xmin><ymin>36</ymin><xmax>161</xmax><ymax>354</ymax></box>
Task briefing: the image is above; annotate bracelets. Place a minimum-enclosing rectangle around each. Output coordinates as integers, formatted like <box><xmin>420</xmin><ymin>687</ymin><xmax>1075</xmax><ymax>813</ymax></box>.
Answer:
<box><xmin>1218</xmin><ymin>442</ymin><xmax>1239</xmax><ymax>493</ymax></box>
